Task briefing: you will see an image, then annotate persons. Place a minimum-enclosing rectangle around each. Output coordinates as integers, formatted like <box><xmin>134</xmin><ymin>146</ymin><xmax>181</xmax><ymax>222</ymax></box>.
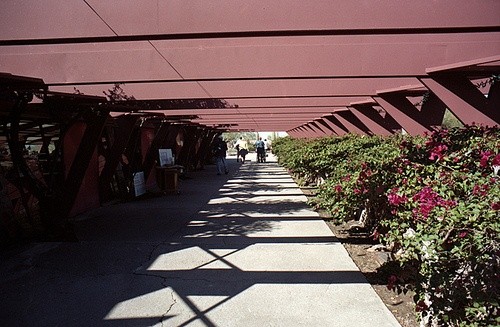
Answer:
<box><xmin>255</xmin><ymin>137</ymin><xmax>265</xmax><ymax>163</ymax></box>
<box><xmin>237</xmin><ymin>137</ymin><xmax>248</xmax><ymax>163</ymax></box>
<box><xmin>216</xmin><ymin>136</ymin><xmax>230</xmax><ymax>176</ymax></box>
